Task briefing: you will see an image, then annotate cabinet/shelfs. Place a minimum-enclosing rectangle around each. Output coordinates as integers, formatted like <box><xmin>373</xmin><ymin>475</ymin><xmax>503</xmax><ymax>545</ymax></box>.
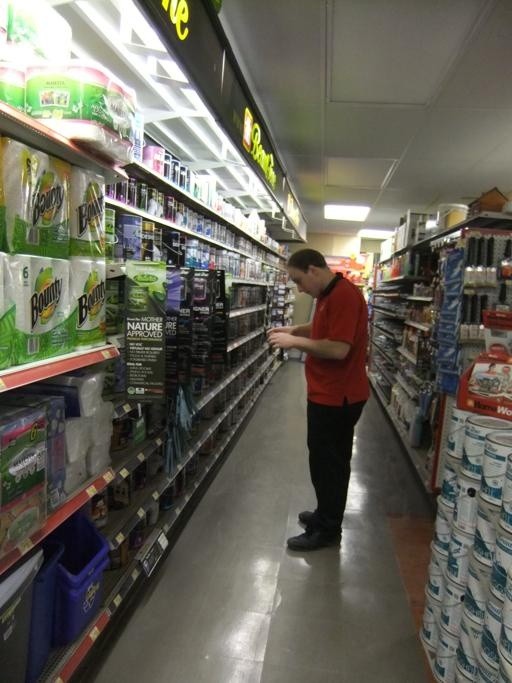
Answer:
<box><xmin>367</xmin><ymin>211</ymin><xmax>512</xmax><ymax>499</ymax></box>
<box><xmin>106</xmin><ymin>161</ymin><xmax>283</xmax><ymax>577</ymax></box>
<box><xmin>1</xmin><ymin>102</ymin><xmax>141</xmax><ymax>683</ymax></box>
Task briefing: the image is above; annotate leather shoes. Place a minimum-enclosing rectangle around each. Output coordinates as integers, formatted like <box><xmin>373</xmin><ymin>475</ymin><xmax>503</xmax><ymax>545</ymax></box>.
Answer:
<box><xmin>286</xmin><ymin>531</ymin><xmax>341</xmax><ymax>551</ymax></box>
<box><xmin>298</xmin><ymin>510</ymin><xmax>313</xmax><ymax>529</ymax></box>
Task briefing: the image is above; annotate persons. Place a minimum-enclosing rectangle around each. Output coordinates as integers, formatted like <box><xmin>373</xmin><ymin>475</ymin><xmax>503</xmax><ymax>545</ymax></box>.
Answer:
<box><xmin>266</xmin><ymin>248</ymin><xmax>371</xmax><ymax>552</ymax></box>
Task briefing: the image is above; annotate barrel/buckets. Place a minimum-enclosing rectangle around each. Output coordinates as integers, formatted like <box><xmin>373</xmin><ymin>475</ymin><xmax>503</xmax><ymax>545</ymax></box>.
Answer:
<box><xmin>432</xmin><ymin>494</ymin><xmax>454</xmax><ymax>557</ymax></box>
<box><xmin>482</xmin><ymin>587</ymin><xmax>504</xmax><ymax>645</ymax></box>
<box><xmin>462</xmin><ymin>565</ymin><xmax>488</xmax><ymax>626</ymax></box>
<box><xmin>498</xmin><ymin>453</ymin><xmax>512</xmax><ymax>535</ymax></box>
<box><xmin>479</xmin><ymin>600</ymin><xmax>503</xmax><ymax>671</ymax></box>
<box><xmin>438</xmin><ymin>569</ymin><xmax>468</xmax><ymax>637</ymax></box>
<box><xmin>425</xmin><ymin>541</ymin><xmax>449</xmax><ymax>602</ymax></box>
<box><xmin>447</xmin><ymin>406</ymin><xmax>478</xmax><ymax>460</ymax></box>
<box><xmin>498</xmin><ymin>623</ymin><xmax>512</xmax><ymax>665</ymax></box>
<box><xmin>460</xmin><ymin>414</ymin><xmax>512</xmax><ymax>481</ymax></box>
<box><xmin>431</xmin><ymin>618</ymin><xmax>460</xmax><ymax>682</ymax></box>
<box><xmin>444</xmin><ymin>521</ymin><xmax>476</xmax><ymax>587</ymax></box>
<box><xmin>451</xmin><ymin>471</ymin><xmax>481</xmax><ymax>536</ymax></box>
<box><xmin>502</xmin><ymin>562</ymin><xmax>512</xmax><ymax>629</ymax></box>
<box><xmin>419</xmin><ymin>586</ymin><xmax>444</xmax><ymax>649</ymax></box>
<box><xmin>498</xmin><ymin>648</ymin><xmax>512</xmax><ymax>683</ymax></box>
<box><xmin>478</xmin><ymin>652</ymin><xmax>499</xmax><ymax>683</ymax></box>
<box><xmin>473</xmin><ymin>507</ymin><xmax>500</xmax><ymax>567</ymax></box>
<box><xmin>488</xmin><ymin>536</ymin><xmax>512</xmax><ymax>602</ymax></box>
<box><xmin>459</xmin><ymin>606</ymin><xmax>483</xmax><ymax>682</ymax></box>
<box><xmin>479</xmin><ymin>430</ymin><xmax>512</xmax><ymax>507</ymax></box>
<box><xmin>464</xmin><ymin>544</ymin><xmax>493</xmax><ymax>602</ymax></box>
<box><xmin>440</xmin><ymin>448</ymin><xmax>464</xmax><ymax>509</ymax></box>
